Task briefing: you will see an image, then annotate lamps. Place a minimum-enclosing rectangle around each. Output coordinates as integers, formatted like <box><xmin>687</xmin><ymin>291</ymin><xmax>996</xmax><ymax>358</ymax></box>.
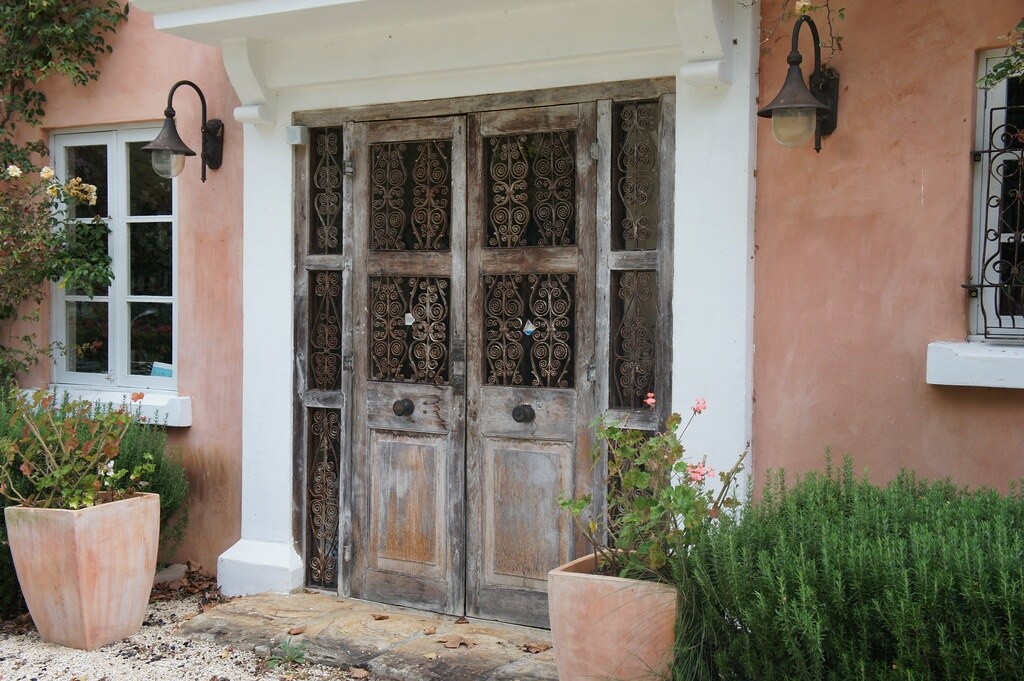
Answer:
<box><xmin>756</xmin><ymin>15</ymin><xmax>840</xmax><ymax>154</ymax></box>
<box><xmin>139</xmin><ymin>80</ymin><xmax>225</xmax><ymax>183</ymax></box>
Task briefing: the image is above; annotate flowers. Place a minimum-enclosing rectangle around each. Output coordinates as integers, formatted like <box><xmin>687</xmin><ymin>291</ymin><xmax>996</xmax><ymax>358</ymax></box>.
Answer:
<box><xmin>0</xmin><ymin>380</ymin><xmax>157</xmax><ymax>510</ymax></box>
<box><xmin>553</xmin><ymin>393</ymin><xmax>751</xmax><ymax>585</ymax></box>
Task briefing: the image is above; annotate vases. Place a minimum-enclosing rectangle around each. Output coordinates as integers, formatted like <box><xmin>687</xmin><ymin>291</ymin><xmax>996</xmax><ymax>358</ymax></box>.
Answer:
<box><xmin>547</xmin><ymin>549</ymin><xmax>688</xmax><ymax>681</ymax></box>
<box><xmin>4</xmin><ymin>491</ymin><xmax>161</xmax><ymax>652</ymax></box>
